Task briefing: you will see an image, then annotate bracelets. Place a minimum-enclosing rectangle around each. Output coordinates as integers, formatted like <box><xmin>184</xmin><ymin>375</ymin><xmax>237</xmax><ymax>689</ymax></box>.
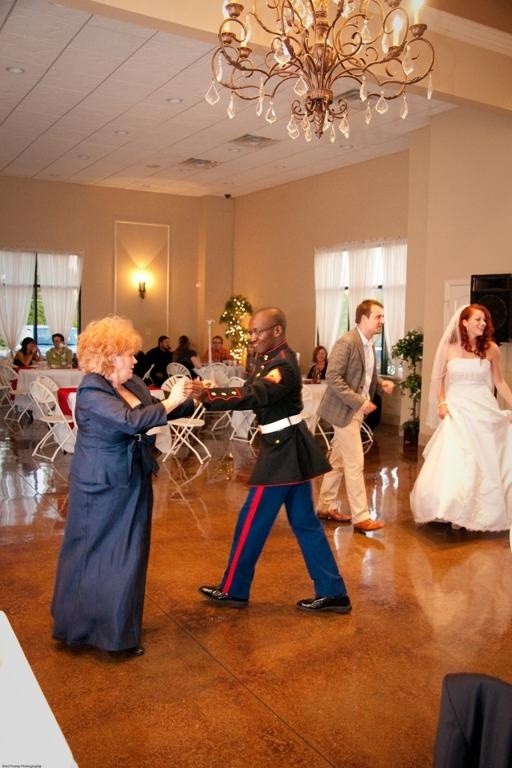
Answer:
<box><xmin>439</xmin><ymin>401</ymin><xmax>446</xmax><ymax>408</ymax></box>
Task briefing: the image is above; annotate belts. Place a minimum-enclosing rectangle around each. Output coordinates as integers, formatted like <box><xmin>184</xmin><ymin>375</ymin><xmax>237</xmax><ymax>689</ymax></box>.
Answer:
<box><xmin>258</xmin><ymin>414</ymin><xmax>303</xmax><ymax>435</ymax></box>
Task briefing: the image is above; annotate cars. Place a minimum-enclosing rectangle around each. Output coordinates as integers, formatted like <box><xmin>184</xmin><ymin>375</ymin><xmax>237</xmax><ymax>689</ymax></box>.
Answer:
<box><xmin>6</xmin><ymin>324</ymin><xmax>78</xmax><ymax>359</ymax></box>
<box><xmin>373</xmin><ymin>334</ymin><xmax>391</xmax><ymax>368</ymax></box>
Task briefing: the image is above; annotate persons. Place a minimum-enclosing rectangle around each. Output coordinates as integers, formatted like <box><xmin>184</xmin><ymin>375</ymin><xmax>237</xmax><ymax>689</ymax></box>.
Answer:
<box><xmin>46</xmin><ymin>334</ymin><xmax>72</xmax><ymax>369</ymax></box>
<box><xmin>307</xmin><ymin>346</ymin><xmax>328</xmax><ymax>379</ymax></box>
<box><xmin>182</xmin><ymin>307</ymin><xmax>352</xmax><ymax>615</ymax></box>
<box><xmin>50</xmin><ymin>316</ymin><xmax>190</xmax><ymax>655</ymax></box>
<box><xmin>408</xmin><ymin>304</ymin><xmax>512</xmax><ymax>532</ymax></box>
<box><xmin>315</xmin><ymin>299</ymin><xmax>394</xmax><ymax>530</ymax></box>
<box><xmin>13</xmin><ymin>337</ymin><xmax>41</xmax><ymax>424</ymax></box>
<box><xmin>133</xmin><ymin>334</ymin><xmax>233</xmax><ymax>386</ymax></box>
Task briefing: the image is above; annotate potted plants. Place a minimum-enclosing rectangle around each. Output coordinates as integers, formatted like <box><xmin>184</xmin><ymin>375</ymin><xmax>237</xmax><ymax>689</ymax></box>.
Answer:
<box><xmin>391</xmin><ymin>327</ymin><xmax>423</xmax><ymax>448</ymax></box>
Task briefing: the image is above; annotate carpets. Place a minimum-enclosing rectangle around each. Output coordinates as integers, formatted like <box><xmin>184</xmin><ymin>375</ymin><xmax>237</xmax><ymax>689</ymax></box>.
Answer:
<box><xmin>0</xmin><ymin>609</ymin><xmax>79</xmax><ymax>768</ymax></box>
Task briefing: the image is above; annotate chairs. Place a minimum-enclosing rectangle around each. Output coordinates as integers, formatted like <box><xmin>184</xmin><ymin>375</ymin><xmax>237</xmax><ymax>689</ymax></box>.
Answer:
<box><xmin>162</xmin><ymin>457</ymin><xmax>213</xmax><ymax>541</ymax></box>
<box><xmin>0</xmin><ymin>361</ymin><xmax>374</xmax><ymax>465</ymax></box>
<box><xmin>433</xmin><ymin>672</ymin><xmax>512</xmax><ymax>767</ymax></box>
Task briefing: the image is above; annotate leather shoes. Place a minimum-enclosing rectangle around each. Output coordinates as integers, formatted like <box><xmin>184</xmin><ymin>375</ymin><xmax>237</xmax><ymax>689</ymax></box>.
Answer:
<box><xmin>318</xmin><ymin>509</ymin><xmax>351</xmax><ymax>522</ymax></box>
<box><xmin>295</xmin><ymin>596</ymin><xmax>352</xmax><ymax>613</ymax></box>
<box><xmin>353</xmin><ymin>518</ymin><xmax>385</xmax><ymax>532</ymax></box>
<box><xmin>198</xmin><ymin>585</ymin><xmax>248</xmax><ymax>609</ymax></box>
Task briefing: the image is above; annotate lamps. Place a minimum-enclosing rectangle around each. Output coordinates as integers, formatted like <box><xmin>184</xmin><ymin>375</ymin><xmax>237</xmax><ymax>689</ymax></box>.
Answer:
<box><xmin>204</xmin><ymin>0</ymin><xmax>435</xmax><ymax>144</ymax></box>
<box><xmin>138</xmin><ymin>281</ymin><xmax>146</xmax><ymax>294</ymax></box>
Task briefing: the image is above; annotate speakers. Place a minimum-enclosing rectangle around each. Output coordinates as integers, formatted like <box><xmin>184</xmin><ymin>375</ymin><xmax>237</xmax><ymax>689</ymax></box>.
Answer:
<box><xmin>470</xmin><ymin>273</ymin><xmax>512</xmax><ymax>342</ymax></box>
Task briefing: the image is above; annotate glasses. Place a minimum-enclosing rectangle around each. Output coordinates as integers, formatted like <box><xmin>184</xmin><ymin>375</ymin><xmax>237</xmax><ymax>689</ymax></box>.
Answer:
<box><xmin>248</xmin><ymin>325</ymin><xmax>276</xmax><ymax>337</ymax></box>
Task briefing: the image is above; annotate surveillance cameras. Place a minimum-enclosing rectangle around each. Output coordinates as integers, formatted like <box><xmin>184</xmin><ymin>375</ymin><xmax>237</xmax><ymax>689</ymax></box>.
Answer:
<box><xmin>224</xmin><ymin>194</ymin><xmax>231</xmax><ymax>199</ymax></box>
